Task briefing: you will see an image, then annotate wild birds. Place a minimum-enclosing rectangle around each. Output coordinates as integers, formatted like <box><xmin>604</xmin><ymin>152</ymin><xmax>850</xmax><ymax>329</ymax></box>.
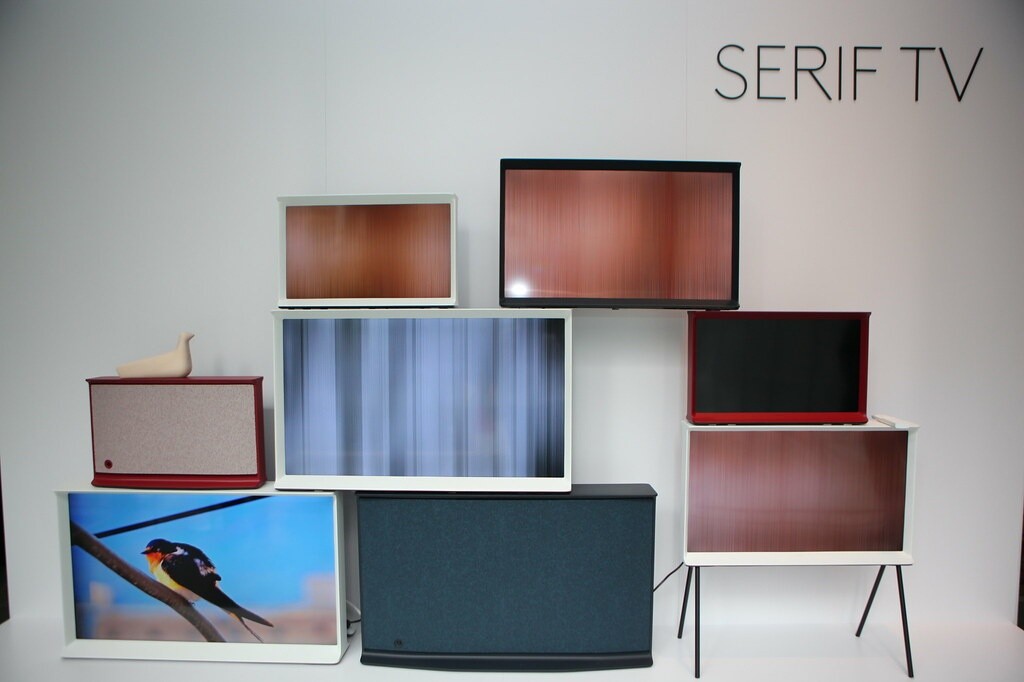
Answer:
<box><xmin>140</xmin><ymin>538</ymin><xmax>274</xmax><ymax>643</ymax></box>
